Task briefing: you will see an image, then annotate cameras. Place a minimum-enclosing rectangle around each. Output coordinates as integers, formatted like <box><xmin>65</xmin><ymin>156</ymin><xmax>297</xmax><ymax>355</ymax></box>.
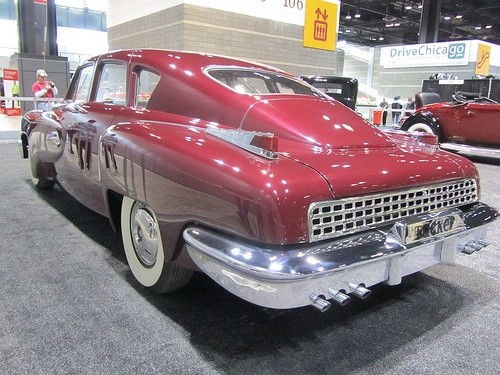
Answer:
<box><xmin>48</xmin><ymin>81</ymin><xmax>54</xmax><ymax>88</ymax></box>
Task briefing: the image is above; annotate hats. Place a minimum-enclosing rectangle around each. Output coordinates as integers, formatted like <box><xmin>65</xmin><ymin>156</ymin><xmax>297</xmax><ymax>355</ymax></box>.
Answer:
<box><xmin>36</xmin><ymin>69</ymin><xmax>47</xmax><ymax>77</ymax></box>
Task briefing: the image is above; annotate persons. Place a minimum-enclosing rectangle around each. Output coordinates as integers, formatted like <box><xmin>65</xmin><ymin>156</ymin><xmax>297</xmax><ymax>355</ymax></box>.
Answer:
<box><xmin>379</xmin><ymin>98</ymin><xmax>389</xmax><ymax>126</ymax></box>
<box><xmin>31</xmin><ymin>69</ymin><xmax>58</xmax><ymax>112</ymax></box>
<box><xmin>11</xmin><ymin>81</ymin><xmax>20</xmax><ymax>108</ymax></box>
<box><xmin>404</xmin><ymin>97</ymin><xmax>415</xmax><ymax>117</ymax></box>
<box><xmin>71</xmin><ymin>119</ymin><xmax>97</xmax><ymax>170</ymax></box>
<box><xmin>66</xmin><ymin>128</ymin><xmax>79</xmax><ymax>156</ymax></box>
<box><xmin>397</xmin><ymin>96</ymin><xmax>404</xmax><ymax>120</ymax></box>
<box><xmin>101</xmin><ymin>133</ymin><xmax>119</xmax><ymax>174</ymax></box>
<box><xmin>391</xmin><ymin>96</ymin><xmax>402</xmax><ymax>127</ymax></box>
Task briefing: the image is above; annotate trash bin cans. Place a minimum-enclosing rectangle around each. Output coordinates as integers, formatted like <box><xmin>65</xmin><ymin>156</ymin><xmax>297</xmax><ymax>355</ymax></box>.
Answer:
<box><xmin>373</xmin><ymin>111</ymin><xmax>382</xmax><ymax>126</ymax></box>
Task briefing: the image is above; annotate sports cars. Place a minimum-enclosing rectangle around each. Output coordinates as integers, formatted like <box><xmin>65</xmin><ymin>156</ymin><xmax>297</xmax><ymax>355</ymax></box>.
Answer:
<box><xmin>395</xmin><ymin>91</ymin><xmax>499</xmax><ymax>161</ymax></box>
<box><xmin>19</xmin><ymin>45</ymin><xmax>496</xmax><ymax>312</ymax></box>
